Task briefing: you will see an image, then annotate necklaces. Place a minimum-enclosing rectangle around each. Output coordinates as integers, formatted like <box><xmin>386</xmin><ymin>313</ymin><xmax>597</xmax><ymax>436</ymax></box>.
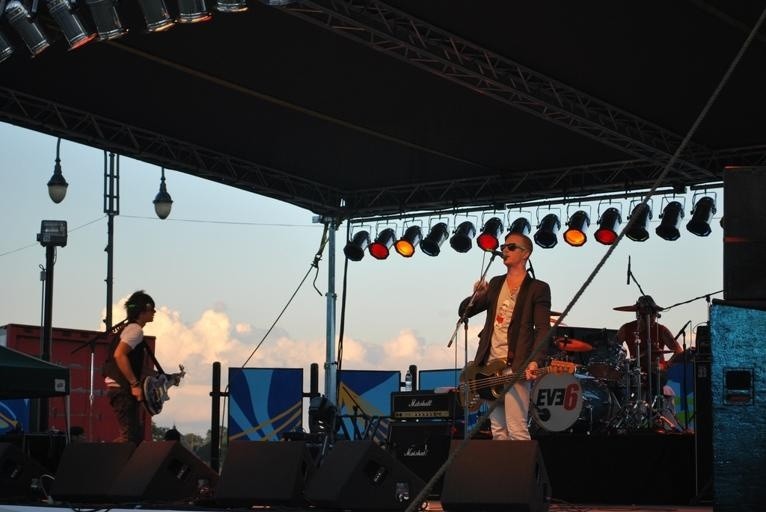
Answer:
<box><xmin>506</xmin><ymin>279</ymin><xmax>522</xmax><ymax>295</ymax></box>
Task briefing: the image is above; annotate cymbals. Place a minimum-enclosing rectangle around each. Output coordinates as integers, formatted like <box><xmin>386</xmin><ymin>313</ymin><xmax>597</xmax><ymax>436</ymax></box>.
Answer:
<box><xmin>644</xmin><ymin>350</ymin><xmax>673</xmax><ymax>354</ymax></box>
<box><xmin>552</xmin><ymin>335</ymin><xmax>592</xmax><ymax>351</ymax></box>
<box><xmin>613</xmin><ymin>305</ymin><xmax>663</xmax><ymax>313</ymax></box>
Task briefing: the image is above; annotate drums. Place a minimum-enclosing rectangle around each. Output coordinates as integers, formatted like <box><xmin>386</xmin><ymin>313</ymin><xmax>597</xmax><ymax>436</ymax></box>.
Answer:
<box><xmin>568</xmin><ymin>374</ymin><xmax>609</xmax><ymax>431</ymax></box>
<box><xmin>585</xmin><ymin>358</ymin><xmax>623</xmax><ymax>384</ymax></box>
<box><xmin>587</xmin><ymin>342</ymin><xmax>628</xmax><ymax>379</ymax></box>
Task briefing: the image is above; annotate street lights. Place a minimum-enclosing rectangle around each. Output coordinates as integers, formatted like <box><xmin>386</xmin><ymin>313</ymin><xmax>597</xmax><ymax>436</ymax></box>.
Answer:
<box><xmin>45</xmin><ymin>133</ymin><xmax>173</xmax><ymax>331</ymax></box>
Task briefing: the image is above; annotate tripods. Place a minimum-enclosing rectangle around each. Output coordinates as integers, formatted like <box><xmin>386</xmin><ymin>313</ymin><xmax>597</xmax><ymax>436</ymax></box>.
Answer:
<box><xmin>593</xmin><ymin>345</ymin><xmax>692</xmax><ymax>434</ymax></box>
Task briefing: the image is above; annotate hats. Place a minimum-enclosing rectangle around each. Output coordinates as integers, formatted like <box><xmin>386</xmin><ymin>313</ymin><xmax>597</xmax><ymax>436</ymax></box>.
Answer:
<box><xmin>636</xmin><ymin>296</ymin><xmax>658</xmax><ymax>315</ymax></box>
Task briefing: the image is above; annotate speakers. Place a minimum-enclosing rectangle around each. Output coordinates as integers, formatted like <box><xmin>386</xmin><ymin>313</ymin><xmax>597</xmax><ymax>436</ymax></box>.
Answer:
<box><xmin>694</xmin><ymin>301</ymin><xmax>765</xmax><ymax>512</ymax></box>
<box><xmin>214</xmin><ymin>440</ymin><xmax>316</xmax><ymax>507</ymax></box>
<box><xmin>724</xmin><ymin>166</ymin><xmax>766</xmax><ymax>301</ymax></box>
<box><xmin>106</xmin><ymin>441</ymin><xmax>219</xmax><ymax>501</ymax></box>
<box><xmin>47</xmin><ymin>443</ymin><xmax>136</xmax><ymax>499</ymax></box>
<box><xmin>440</xmin><ymin>440</ymin><xmax>553</xmax><ymax>512</ymax></box>
<box><xmin>305</xmin><ymin>439</ymin><xmax>427</xmax><ymax>512</ymax></box>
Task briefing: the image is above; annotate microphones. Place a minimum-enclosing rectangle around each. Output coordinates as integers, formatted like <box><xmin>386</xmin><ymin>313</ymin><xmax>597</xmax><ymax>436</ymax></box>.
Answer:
<box><xmin>491</xmin><ymin>250</ymin><xmax>507</xmax><ymax>260</ymax></box>
<box><xmin>673</xmin><ymin>321</ymin><xmax>690</xmax><ymax>341</ymax></box>
<box><xmin>627</xmin><ymin>256</ymin><xmax>630</xmax><ymax>284</ymax></box>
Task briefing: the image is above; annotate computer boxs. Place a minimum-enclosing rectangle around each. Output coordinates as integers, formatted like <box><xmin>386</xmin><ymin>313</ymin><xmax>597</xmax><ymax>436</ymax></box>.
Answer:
<box><xmin>24</xmin><ymin>432</ymin><xmax>68</xmax><ymax>473</ymax></box>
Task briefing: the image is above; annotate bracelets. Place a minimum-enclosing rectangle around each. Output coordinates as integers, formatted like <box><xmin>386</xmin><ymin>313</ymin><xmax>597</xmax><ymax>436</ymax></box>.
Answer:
<box><xmin>130</xmin><ymin>380</ymin><xmax>141</xmax><ymax>388</ymax></box>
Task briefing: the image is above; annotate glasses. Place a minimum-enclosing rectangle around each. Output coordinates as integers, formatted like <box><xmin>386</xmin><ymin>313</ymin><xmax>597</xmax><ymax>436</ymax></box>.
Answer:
<box><xmin>500</xmin><ymin>242</ymin><xmax>526</xmax><ymax>249</ymax></box>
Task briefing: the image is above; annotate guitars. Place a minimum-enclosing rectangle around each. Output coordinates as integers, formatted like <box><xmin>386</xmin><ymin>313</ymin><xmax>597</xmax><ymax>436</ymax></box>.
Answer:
<box><xmin>141</xmin><ymin>364</ymin><xmax>186</xmax><ymax>415</ymax></box>
<box><xmin>455</xmin><ymin>359</ymin><xmax>576</xmax><ymax>410</ymax></box>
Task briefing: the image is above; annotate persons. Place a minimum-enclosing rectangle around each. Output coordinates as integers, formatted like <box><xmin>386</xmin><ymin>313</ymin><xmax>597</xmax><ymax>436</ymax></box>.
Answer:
<box><xmin>70</xmin><ymin>425</ymin><xmax>84</xmax><ymax>442</ymax></box>
<box><xmin>158</xmin><ymin>425</ymin><xmax>200</xmax><ymax>500</ymax></box>
<box><xmin>103</xmin><ymin>291</ymin><xmax>155</xmax><ymax>448</ymax></box>
<box><xmin>616</xmin><ymin>299</ymin><xmax>682</xmax><ymax>434</ymax></box>
<box><xmin>458</xmin><ymin>234</ymin><xmax>551</xmax><ymax>440</ymax></box>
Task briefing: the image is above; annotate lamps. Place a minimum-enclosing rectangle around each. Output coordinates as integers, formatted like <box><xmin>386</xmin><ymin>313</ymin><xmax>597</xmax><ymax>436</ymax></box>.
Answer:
<box><xmin>507</xmin><ymin>188</ymin><xmax>685</xmax><ymax>251</ymax></box>
<box><xmin>687</xmin><ymin>182</ymin><xmax>724</xmax><ymax>239</ymax></box>
<box><xmin>47</xmin><ymin>134</ymin><xmax>70</xmax><ymax>204</ymax></box>
<box><xmin>1</xmin><ymin>1</ymin><xmax>254</xmax><ymax>62</ymax></box>
<box><xmin>153</xmin><ymin>167</ymin><xmax>175</xmax><ymax>221</ymax></box>
<box><xmin>343</xmin><ymin>202</ymin><xmax>506</xmax><ymax>261</ymax></box>
